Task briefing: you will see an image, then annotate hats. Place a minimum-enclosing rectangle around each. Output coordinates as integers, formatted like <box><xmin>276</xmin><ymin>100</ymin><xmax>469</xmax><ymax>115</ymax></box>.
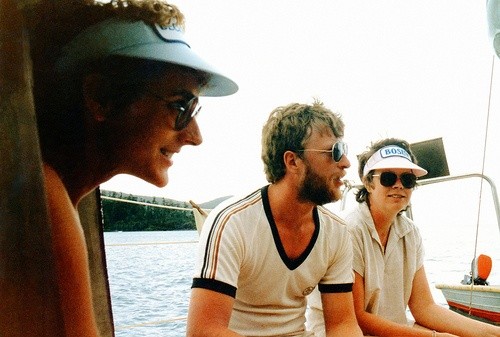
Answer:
<box><xmin>48</xmin><ymin>15</ymin><xmax>239</xmax><ymax>97</ymax></box>
<box><xmin>363</xmin><ymin>145</ymin><xmax>428</xmax><ymax>178</ymax></box>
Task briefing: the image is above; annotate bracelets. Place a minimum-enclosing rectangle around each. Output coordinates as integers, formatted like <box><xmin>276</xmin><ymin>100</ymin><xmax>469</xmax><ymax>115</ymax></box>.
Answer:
<box><xmin>432</xmin><ymin>330</ymin><xmax>437</xmax><ymax>337</ymax></box>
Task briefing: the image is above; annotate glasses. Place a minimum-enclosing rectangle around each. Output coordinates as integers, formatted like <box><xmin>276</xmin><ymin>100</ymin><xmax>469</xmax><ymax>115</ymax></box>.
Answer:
<box><xmin>372</xmin><ymin>172</ymin><xmax>417</xmax><ymax>188</ymax></box>
<box><xmin>103</xmin><ymin>70</ymin><xmax>198</xmax><ymax>131</ymax></box>
<box><xmin>298</xmin><ymin>141</ymin><xmax>348</xmax><ymax>162</ymax></box>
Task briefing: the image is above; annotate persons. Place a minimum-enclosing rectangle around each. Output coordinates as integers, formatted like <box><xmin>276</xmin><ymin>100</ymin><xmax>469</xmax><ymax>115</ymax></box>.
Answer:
<box><xmin>186</xmin><ymin>98</ymin><xmax>365</xmax><ymax>337</ymax></box>
<box><xmin>307</xmin><ymin>139</ymin><xmax>500</xmax><ymax>337</ymax></box>
<box><xmin>31</xmin><ymin>0</ymin><xmax>238</xmax><ymax>337</ymax></box>
